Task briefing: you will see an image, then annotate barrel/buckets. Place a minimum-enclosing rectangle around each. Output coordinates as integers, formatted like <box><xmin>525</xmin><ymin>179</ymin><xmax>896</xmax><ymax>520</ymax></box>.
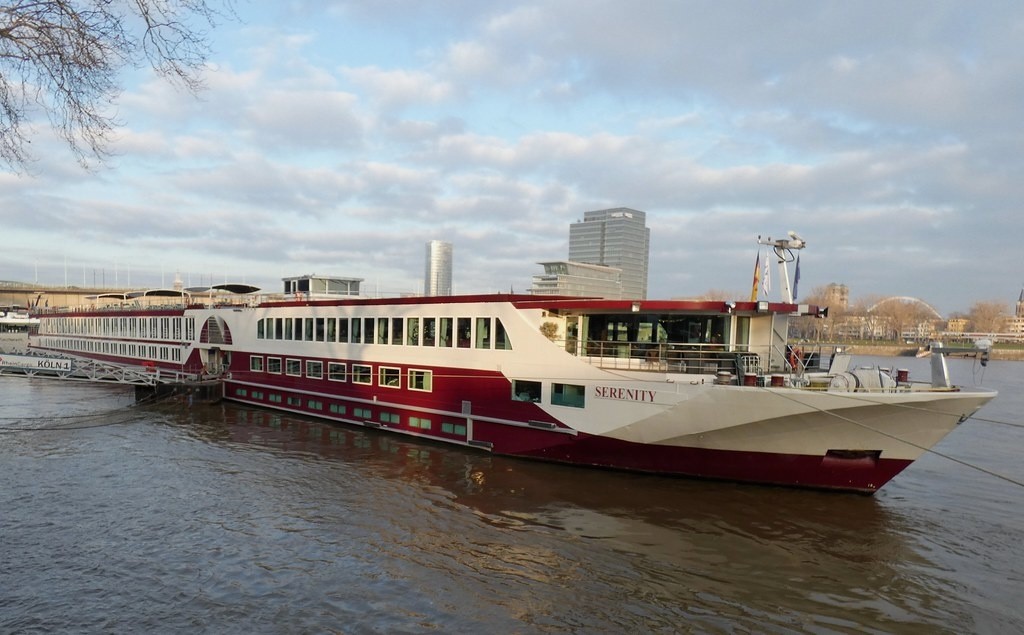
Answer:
<box><xmin>771</xmin><ymin>376</ymin><xmax>785</xmax><ymax>386</ymax></box>
<box><xmin>718</xmin><ymin>372</ymin><xmax>731</xmax><ymax>385</ymax></box>
<box><xmin>880</xmin><ymin>368</ymin><xmax>890</xmax><ymax>372</ymax></box>
<box><xmin>744</xmin><ymin>374</ymin><xmax>756</xmax><ymax>386</ymax></box>
<box><xmin>756</xmin><ymin>376</ymin><xmax>765</xmax><ymax>387</ymax></box>
<box><xmin>897</xmin><ymin>369</ymin><xmax>909</xmax><ymax>381</ymax></box>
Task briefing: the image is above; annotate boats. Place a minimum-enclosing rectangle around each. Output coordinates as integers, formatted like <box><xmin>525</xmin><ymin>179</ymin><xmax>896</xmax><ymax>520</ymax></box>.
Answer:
<box><xmin>0</xmin><ymin>233</ymin><xmax>1000</xmax><ymax>498</ymax></box>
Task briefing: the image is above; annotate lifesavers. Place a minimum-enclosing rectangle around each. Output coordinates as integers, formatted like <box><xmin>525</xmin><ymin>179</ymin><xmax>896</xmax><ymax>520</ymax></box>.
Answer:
<box><xmin>295</xmin><ymin>291</ymin><xmax>302</xmax><ymax>301</ymax></box>
<box><xmin>790</xmin><ymin>348</ymin><xmax>802</xmax><ymax>368</ymax></box>
<box><xmin>186</xmin><ymin>298</ymin><xmax>190</xmax><ymax>306</ymax></box>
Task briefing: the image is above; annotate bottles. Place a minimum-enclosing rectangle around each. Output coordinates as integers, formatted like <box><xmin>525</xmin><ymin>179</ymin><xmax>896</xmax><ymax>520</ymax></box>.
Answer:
<box><xmin>679</xmin><ymin>361</ymin><xmax>686</xmax><ymax>373</ymax></box>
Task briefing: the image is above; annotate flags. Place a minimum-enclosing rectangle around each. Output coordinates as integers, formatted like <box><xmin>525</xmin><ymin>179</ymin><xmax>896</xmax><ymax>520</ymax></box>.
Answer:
<box><xmin>793</xmin><ymin>255</ymin><xmax>800</xmax><ymax>300</ymax></box>
<box><xmin>751</xmin><ymin>253</ymin><xmax>760</xmax><ymax>302</ymax></box>
<box><xmin>762</xmin><ymin>252</ymin><xmax>771</xmax><ymax>296</ymax></box>
